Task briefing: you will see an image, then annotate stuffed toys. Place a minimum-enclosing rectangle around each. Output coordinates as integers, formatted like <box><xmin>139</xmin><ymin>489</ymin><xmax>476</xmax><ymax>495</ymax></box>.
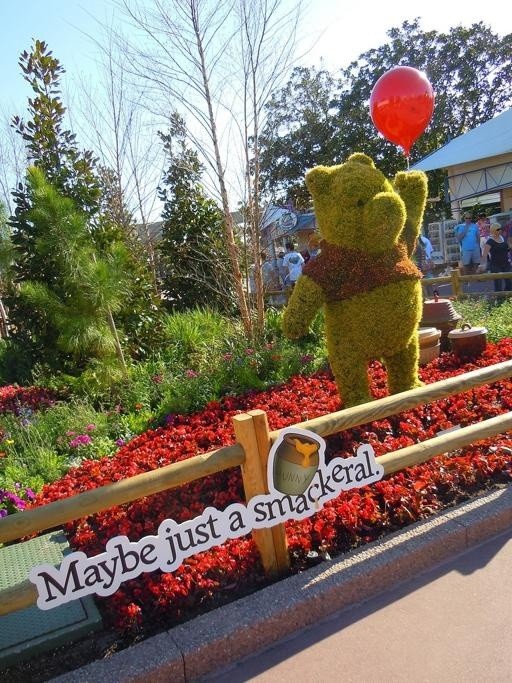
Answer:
<box><xmin>278</xmin><ymin>147</ymin><xmax>428</xmax><ymax>409</ymax></box>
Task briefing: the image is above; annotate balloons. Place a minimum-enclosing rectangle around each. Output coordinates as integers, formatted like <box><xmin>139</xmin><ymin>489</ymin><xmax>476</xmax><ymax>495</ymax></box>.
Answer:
<box><xmin>368</xmin><ymin>64</ymin><xmax>435</xmax><ymax>158</ymax></box>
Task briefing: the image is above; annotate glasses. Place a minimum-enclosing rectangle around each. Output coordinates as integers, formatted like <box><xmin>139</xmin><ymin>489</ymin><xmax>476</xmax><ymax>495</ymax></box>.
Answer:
<box><xmin>465</xmin><ymin>217</ymin><xmax>471</xmax><ymax>219</ymax></box>
<box><xmin>496</xmin><ymin>228</ymin><xmax>501</xmax><ymax>231</ymax></box>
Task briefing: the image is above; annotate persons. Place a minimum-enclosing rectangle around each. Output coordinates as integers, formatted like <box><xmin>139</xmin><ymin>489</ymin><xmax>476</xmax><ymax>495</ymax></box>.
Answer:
<box><xmin>456</xmin><ymin>211</ymin><xmax>511</xmax><ymax>280</ymax></box>
<box><xmin>478</xmin><ymin>222</ymin><xmax>511</xmax><ymax>293</ymax></box>
<box><xmin>250</xmin><ymin>237</ymin><xmax>314</xmax><ymax>297</ymax></box>
<box><xmin>416</xmin><ymin>232</ymin><xmax>433</xmax><ymax>264</ymax></box>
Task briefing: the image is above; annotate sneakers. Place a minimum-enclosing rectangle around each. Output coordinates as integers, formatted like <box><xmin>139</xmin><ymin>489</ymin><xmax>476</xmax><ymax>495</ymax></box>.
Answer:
<box><xmin>433</xmin><ymin>291</ymin><xmax>439</xmax><ymax>296</ymax></box>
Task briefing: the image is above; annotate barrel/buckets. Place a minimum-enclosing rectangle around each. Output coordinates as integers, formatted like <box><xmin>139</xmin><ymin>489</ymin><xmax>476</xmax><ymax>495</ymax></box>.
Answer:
<box><xmin>449</xmin><ymin>326</ymin><xmax>487</xmax><ymax>362</ymax></box>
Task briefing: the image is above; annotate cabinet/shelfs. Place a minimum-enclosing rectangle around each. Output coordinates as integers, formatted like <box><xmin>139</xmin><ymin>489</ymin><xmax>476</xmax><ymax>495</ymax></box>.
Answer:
<box><xmin>428</xmin><ymin>219</ymin><xmax>460</xmax><ymax>267</ymax></box>
